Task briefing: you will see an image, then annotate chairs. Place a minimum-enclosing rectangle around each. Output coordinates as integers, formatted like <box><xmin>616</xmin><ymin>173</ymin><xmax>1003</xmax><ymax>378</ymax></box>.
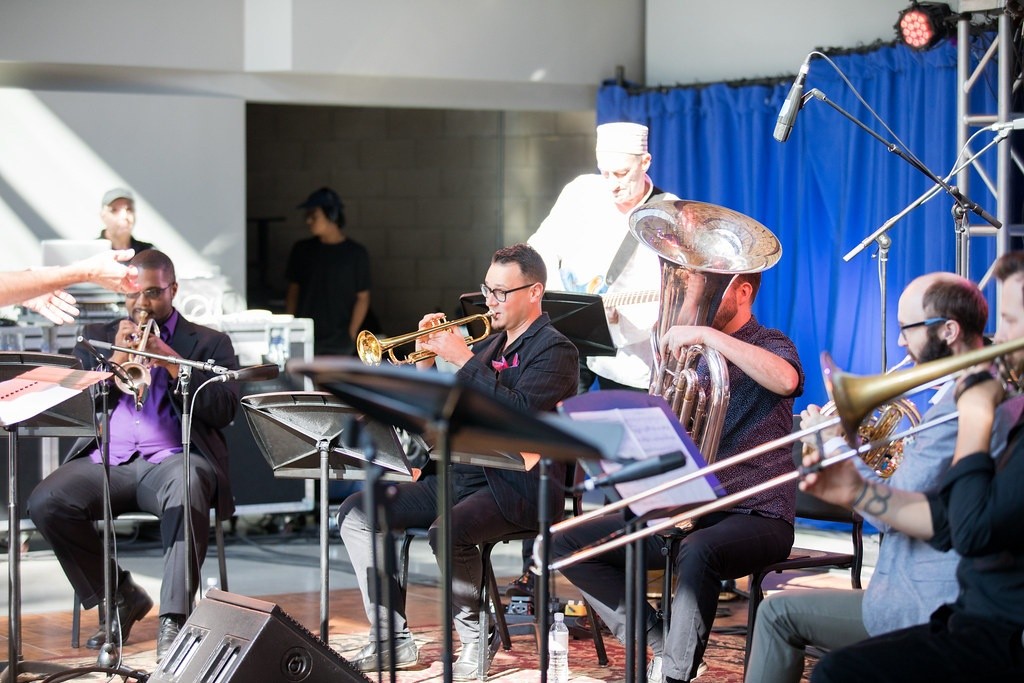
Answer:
<box><xmin>71</xmin><ymin>507</ymin><xmax>229</xmax><ymax>649</ymax></box>
<box><xmin>398</xmin><ymin>457</ymin><xmax>586</xmax><ymax>652</ymax></box>
<box><xmin>583</xmin><ymin>414</ymin><xmax>863</xmax><ymax>683</ymax></box>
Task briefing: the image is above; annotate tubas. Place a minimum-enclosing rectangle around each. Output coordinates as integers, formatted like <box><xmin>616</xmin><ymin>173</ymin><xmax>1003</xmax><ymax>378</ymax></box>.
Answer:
<box><xmin>624</xmin><ymin>197</ymin><xmax>787</xmax><ymax>534</ymax></box>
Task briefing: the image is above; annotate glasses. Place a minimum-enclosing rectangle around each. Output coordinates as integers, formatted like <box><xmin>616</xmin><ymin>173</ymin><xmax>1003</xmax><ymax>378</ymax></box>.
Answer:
<box><xmin>125</xmin><ymin>283</ymin><xmax>174</xmax><ymax>299</ymax></box>
<box><xmin>479</xmin><ymin>281</ymin><xmax>535</xmax><ymax>303</ymax></box>
<box><xmin>898</xmin><ymin>318</ymin><xmax>951</xmax><ymax>342</ymax></box>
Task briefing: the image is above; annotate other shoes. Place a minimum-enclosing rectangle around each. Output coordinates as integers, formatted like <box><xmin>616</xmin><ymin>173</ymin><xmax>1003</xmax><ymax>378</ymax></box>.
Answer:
<box><xmin>503</xmin><ymin>576</ymin><xmax>551</xmax><ymax>605</ymax></box>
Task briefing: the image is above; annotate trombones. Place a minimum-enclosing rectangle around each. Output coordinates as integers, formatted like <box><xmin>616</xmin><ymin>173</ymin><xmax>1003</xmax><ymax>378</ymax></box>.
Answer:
<box><xmin>530</xmin><ymin>338</ymin><xmax>1023</xmax><ymax>578</ymax></box>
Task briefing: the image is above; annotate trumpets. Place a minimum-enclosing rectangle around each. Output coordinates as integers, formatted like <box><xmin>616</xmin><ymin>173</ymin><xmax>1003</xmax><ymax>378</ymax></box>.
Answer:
<box><xmin>114</xmin><ymin>310</ymin><xmax>162</xmax><ymax>397</ymax></box>
<box><xmin>356</xmin><ymin>307</ymin><xmax>501</xmax><ymax>367</ymax></box>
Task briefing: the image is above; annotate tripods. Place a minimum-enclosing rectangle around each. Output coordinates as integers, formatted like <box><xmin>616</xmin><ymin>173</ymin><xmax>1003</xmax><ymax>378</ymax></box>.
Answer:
<box><xmin>0</xmin><ymin>336</ymin><xmax>226</xmax><ymax>683</ymax></box>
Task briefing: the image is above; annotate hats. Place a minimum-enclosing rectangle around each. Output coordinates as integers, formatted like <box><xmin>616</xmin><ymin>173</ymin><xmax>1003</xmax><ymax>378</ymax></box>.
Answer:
<box><xmin>102</xmin><ymin>189</ymin><xmax>135</xmax><ymax>207</ymax></box>
<box><xmin>596</xmin><ymin>122</ymin><xmax>648</xmax><ymax>155</ymax></box>
<box><xmin>297</xmin><ymin>188</ymin><xmax>347</xmax><ymax>217</ymax></box>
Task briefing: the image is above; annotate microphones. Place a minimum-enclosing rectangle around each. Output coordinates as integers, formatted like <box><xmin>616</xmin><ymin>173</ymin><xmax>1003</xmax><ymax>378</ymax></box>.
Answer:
<box><xmin>574</xmin><ymin>450</ymin><xmax>687</xmax><ymax>493</ymax></box>
<box><xmin>136</xmin><ymin>382</ymin><xmax>148</xmax><ymax>411</ymax></box>
<box><xmin>773</xmin><ymin>54</ymin><xmax>811</xmax><ymax>142</ymax></box>
<box><xmin>211</xmin><ymin>363</ymin><xmax>279</xmax><ymax>383</ymax></box>
<box><xmin>990</xmin><ymin>119</ymin><xmax>1024</xmax><ymax>130</ymax></box>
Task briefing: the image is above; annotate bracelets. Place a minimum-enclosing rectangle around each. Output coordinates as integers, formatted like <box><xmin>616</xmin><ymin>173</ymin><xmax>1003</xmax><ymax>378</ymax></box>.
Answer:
<box><xmin>852</xmin><ymin>481</ymin><xmax>870</xmax><ymax>508</ymax></box>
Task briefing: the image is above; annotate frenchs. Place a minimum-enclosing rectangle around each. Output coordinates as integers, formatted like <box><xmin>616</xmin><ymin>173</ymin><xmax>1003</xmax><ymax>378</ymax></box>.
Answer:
<box><xmin>800</xmin><ymin>354</ymin><xmax>923</xmax><ymax>480</ymax></box>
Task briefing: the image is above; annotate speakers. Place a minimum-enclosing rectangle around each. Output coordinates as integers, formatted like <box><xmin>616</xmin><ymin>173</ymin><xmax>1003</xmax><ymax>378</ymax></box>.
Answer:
<box><xmin>146</xmin><ymin>588</ymin><xmax>375</xmax><ymax>683</ymax></box>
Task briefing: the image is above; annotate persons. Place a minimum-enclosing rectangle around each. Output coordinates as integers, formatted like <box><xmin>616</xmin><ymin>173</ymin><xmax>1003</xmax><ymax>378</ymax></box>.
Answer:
<box><xmin>504</xmin><ymin>122</ymin><xmax>682</xmax><ymax>603</ymax></box>
<box><xmin>285</xmin><ymin>186</ymin><xmax>371</xmax><ymax>356</ymax></box>
<box><xmin>744</xmin><ymin>271</ymin><xmax>1024</xmax><ymax>683</ymax></box>
<box><xmin>0</xmin><ymin>248</ymin><xmax>140</xmax><ymax>326</ymax></box>
<box><xmin>551</xmin><ymin>272</ymin><xmax>806</xmax><ymax>683</ymax></box>
<box><xmin>96</xmin><ymin>188</ymin><xmax>159</xmax><ymax>268</ymax></box>
<box><xmin>335</xmin><ymin>242</ymin><xmax>579</xmax><ymax>683</ymax></box>
<box><xmin>798</xmin><ymin>250</ymin><xmax>1024</xmax><ymax>683</ymax></box>
<box><xmin>27</xmin><ymin>249</ymin><xmax>240</xmax><ymax>664</ymax></box>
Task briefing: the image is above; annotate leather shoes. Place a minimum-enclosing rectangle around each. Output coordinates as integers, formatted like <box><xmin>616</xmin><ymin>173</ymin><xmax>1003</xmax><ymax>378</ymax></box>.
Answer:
<box><xmin>156</xmin><ymin>612</ymin><xmax>184</xmax><ymax>667</ymax></box>
<box><xmin>86</xmin><ymin>570</ymin><xmax>153</xmax><ymax>652</ymax></box>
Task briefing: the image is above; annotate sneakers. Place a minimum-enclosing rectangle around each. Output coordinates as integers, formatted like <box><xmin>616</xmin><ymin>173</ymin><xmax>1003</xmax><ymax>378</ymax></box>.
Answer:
<box><xmin>349</xmin><ymin>637</ymin><xmax>420</xmax><ymax>673</ymax></box>
<box><xmin>645</xmin><ymin>649</ymin><xmax>708</xmax><ymax>683</ymax></box>
<box><xmin>452</xmin><ymin>625</ymin><xmax>501</xmax><ymax>682</ymax></box>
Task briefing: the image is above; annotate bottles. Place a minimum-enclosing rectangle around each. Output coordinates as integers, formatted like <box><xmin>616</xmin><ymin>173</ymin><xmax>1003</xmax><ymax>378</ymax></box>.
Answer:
<box><xmin>549</xmin><ymin>613</ymin><xmax>569</xmax><ymax>683</ymax></box>
<box><xmin>565</xmin><ymin>600</ymin><xmax>586</xmax><ymax>616</ymax></box>
<box><xmin>205</xmin><ymin>578</ymin><xmax>219</xmax><ymax>598</ymax></box>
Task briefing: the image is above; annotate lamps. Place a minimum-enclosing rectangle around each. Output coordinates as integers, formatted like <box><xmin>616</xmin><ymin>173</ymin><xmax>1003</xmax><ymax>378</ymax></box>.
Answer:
<box><xmin>893</xmin><ymin>2</ymin><xmax>953</xmax><ymax>53</ymax></box>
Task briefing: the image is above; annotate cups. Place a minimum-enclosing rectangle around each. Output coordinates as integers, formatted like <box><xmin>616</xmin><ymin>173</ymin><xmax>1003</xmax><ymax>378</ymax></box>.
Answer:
<box><xmin>42</xmin><ymin>326</ymin><xmax>59</xmax><ymax>354</ymax></box>
<box><xmin>0</xmin><ymin>332</ymin><xmax>24</xmax><ymax>351</ymax></box>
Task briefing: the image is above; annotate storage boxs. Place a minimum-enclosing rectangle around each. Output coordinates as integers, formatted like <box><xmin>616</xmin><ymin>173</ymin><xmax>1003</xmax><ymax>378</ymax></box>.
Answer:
<box><xmin>49</xmin><ymin>317</ymin><xmax>316</xmax><ymax>522</ymax></box>
<box><xmin>0</xmin><ymin>326</ymin><xmax>50</xmax><ymax>533</ymax></box>
